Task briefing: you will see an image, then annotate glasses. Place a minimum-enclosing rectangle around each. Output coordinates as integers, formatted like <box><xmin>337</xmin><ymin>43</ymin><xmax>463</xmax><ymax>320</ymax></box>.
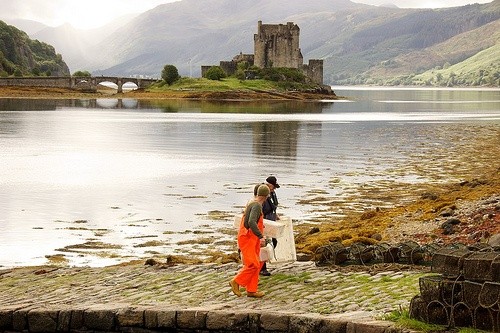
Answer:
<box><xmin>258</xmin><ymin>194</ymin><xmax>271</xmax><ymax>197</ymax></box>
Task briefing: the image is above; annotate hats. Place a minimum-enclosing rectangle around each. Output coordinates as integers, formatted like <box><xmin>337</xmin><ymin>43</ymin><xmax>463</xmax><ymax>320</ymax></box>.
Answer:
<box><xmin>266</xmin><ymin>176</ymin><xmax>280</xmax><ymax>188</ymax></box>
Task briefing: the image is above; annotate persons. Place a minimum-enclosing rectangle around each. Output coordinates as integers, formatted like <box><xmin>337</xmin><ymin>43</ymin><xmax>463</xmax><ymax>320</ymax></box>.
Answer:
<box><xmin>228</xmin><ymin>175</ymin><xmax>279</xmax><ymax>299</ymax></box>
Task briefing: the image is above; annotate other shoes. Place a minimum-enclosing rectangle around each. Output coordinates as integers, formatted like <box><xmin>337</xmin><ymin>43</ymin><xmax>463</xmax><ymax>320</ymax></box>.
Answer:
<box><xmin>247</xmin><ymin>291</ymin><xmax>265</xmax><ymax>297</ymax></box>
<box><xmin>259</xmin><ymin>270</ymin><xmax>271</xmax><ymax>276</ymax></box>
<box><xmin>229</xmin><ymin>279</ymin><xmax>241</xmax><ymax>297</ymax></box>
